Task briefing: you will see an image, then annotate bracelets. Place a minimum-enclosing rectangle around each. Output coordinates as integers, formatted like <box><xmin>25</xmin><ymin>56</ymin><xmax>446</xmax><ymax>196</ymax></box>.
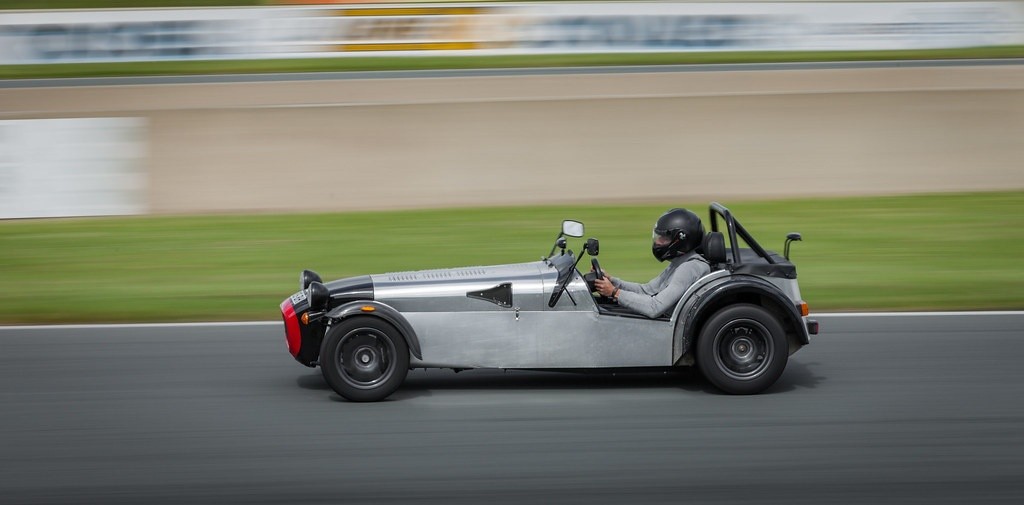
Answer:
<box><xmin>611</xmin><ymin>287</ymin><xmax>618</xmax><ymax>298</ymax></box>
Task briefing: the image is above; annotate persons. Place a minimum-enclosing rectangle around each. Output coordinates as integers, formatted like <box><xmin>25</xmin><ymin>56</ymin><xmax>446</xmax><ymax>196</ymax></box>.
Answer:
<box><xmin>594</xmin><ymin>207</ymin><xmax>711</xmax><ymax>321</ymax></box>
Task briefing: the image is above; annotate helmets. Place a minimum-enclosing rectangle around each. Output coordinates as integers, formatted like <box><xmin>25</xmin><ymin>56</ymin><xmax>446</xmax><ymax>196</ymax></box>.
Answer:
<box><xmin>651</xmin><ymin>208</ymin><xmax>706</xmax><ymax>263</ymax></box>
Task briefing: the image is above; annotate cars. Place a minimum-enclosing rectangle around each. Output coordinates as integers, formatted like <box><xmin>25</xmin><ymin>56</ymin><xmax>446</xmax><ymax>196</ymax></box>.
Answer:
<box><xmin>278</xmin><ymin>202</ymin><xmax>820</xmax><ymax>403</ymax></box>
